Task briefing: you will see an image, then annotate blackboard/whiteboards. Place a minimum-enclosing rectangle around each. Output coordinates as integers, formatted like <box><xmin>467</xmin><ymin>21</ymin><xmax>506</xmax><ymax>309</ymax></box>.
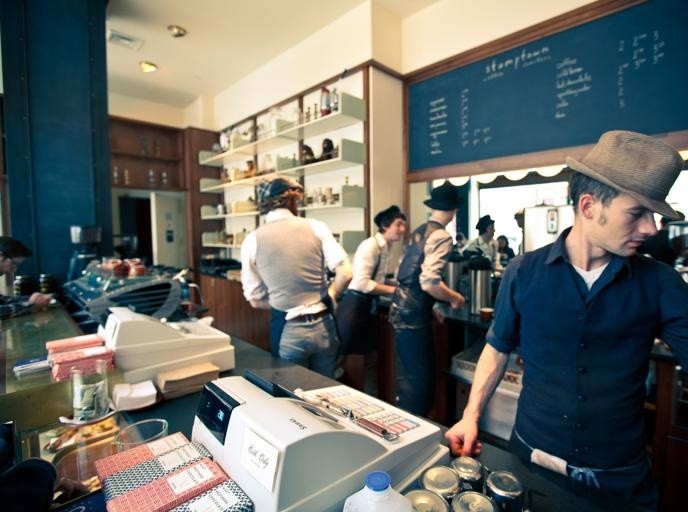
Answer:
<box><xmin>403</xmin><ymin>0</ymin><xmax>687</xmax><ymax>181</ymax></box>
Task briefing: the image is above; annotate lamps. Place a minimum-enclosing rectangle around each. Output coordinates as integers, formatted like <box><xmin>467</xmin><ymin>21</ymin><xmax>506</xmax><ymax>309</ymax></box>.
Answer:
<box><xmin>140</xmin><ymin>62</ymin><xmax>156</xmax><ymax>74</ymax></box>
<box><xmin>167</xmin><ymin>26</ymin><xmax>185</xmax><ymax>37</ymax></box>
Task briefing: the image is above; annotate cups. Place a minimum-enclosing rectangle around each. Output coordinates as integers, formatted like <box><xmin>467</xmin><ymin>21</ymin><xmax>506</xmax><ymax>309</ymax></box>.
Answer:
<box><xmin>71</xmin><ymin>361</ymin><xmax>105</xmax><ymax>417</ymax></box>
<box><xmin>108</xmin><ymin>418</ymin><xmax>168</xmax><ymax>455</ymax></box>
<box><xmin>478</xmin><ymin>307</ymin><xmax>493</xmax><ymax>321</ymax></box>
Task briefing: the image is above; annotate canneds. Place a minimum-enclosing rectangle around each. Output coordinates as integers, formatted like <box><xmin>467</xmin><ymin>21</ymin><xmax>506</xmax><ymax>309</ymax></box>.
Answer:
<box><xmin>403</xmin><ymin>489</ymin><xmax>451</xmax><ymax>512</ymax></box>
<box><xmin>450</xmin><ymin>456</ymin><xmax>485</xmax><ymax>494</ymax></box>
<box><xmin>12</xmin><ymin>274</ymin><xmax>32</xmax><ymax>296</ymax></box>
<box><xmin>451</xmin><ymin>490</ymin><xmax>499</xmax><ymax>512</ymax></box>
<box><xmin>485</xmin><ymin>469</ymin><xmax>523</xmax><ymax>512</ymax></box>
<box><xmin>38</xmin><ymin>273</ymin><xmax>54</xmax><ymax>294</ymax></box>
<box><xmin>418</xmin><ymin>463</ymin><xmax>460</xmax><ymax>500</ymax></box>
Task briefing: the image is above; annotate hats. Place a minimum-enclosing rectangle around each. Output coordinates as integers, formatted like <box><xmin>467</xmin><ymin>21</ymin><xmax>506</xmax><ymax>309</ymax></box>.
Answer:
<box><xmin>259</xmin><ymin>176</ymin><xmax>304</xmax><ymax>202</ymax></box>
<box><xmin>423</xmin><ymin>181</ymin><xmax>465</xmax><ymax>210</ymax></box>
<box><xmin>475</xmin><ymin>215</ymin><xmax>494</xmax><ymax>230</ymax></box>
<box><xmin>565</xmin><ymin>128</ymin><xmax>685</xmax><ymax>221</ymax></box>
<box><xmin>374</xmin><ymin>206</ymin><xmax>400</xmax><ymax>226</ymax></box>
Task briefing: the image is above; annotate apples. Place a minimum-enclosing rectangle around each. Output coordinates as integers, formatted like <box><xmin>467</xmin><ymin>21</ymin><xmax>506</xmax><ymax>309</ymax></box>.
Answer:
<box><xmin>129</xmin><ymin>262</ymin><xmax>146</xmax><ymax>278</ymax></box>
<box><xmin>114</xmin><ymin>262</ymin><xmax>130</xmax><ymax>278</ymax></box>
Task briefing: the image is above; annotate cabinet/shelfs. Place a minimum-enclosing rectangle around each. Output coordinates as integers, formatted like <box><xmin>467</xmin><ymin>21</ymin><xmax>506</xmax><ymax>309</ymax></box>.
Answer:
<box><xmin>198</xmin><ymin>91</ymin><xmax>367</xmax><ymax>260</ymax></box>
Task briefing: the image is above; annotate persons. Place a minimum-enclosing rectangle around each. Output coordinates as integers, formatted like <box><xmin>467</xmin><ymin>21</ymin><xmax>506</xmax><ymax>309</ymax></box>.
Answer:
<box><xmin>388</xmin><ymin>180</ymin><xmax>468</xmax><ymax>417</ymax></box>
<box><xmin>334</xmin><ymin>204</ymin><xmax>410</xmax><ymax>399</ymax></box>
<box><xmin>239</xmin><ymin>177</ymin><xmax>355</xmax><ymax>380</ymax></box>
<box><xmin>442</xmin><ymin>129</ymin><xmax>688</xmax><ymax>511</ymax></box>
<box><xmin>453</xmin><ymin>209</ymin><xmax>688</xmax><ymax>284</ymax></box>
<box><xmin>0</xmin><ymin>234</ymin><xmax>55</xmax><ymax>320</ymax></box>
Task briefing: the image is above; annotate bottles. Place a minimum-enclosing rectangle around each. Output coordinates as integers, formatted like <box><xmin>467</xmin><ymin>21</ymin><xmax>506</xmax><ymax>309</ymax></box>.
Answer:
<box><xmin>12</xmin><ymin>274</ymin><xmax>22</xmax><ymax>296</ymax></box>
<box><xmin>39</xmin><ymin>274</ymin><xmax>48</xmax><ymax>293</ymax></box>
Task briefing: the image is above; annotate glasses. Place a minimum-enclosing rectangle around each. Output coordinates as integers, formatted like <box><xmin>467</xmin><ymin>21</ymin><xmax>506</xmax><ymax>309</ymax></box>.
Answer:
<box><xmin>10</xmin><ymin>258</ymin><xmax>18</xmax><ymax>271</ymax></box>
